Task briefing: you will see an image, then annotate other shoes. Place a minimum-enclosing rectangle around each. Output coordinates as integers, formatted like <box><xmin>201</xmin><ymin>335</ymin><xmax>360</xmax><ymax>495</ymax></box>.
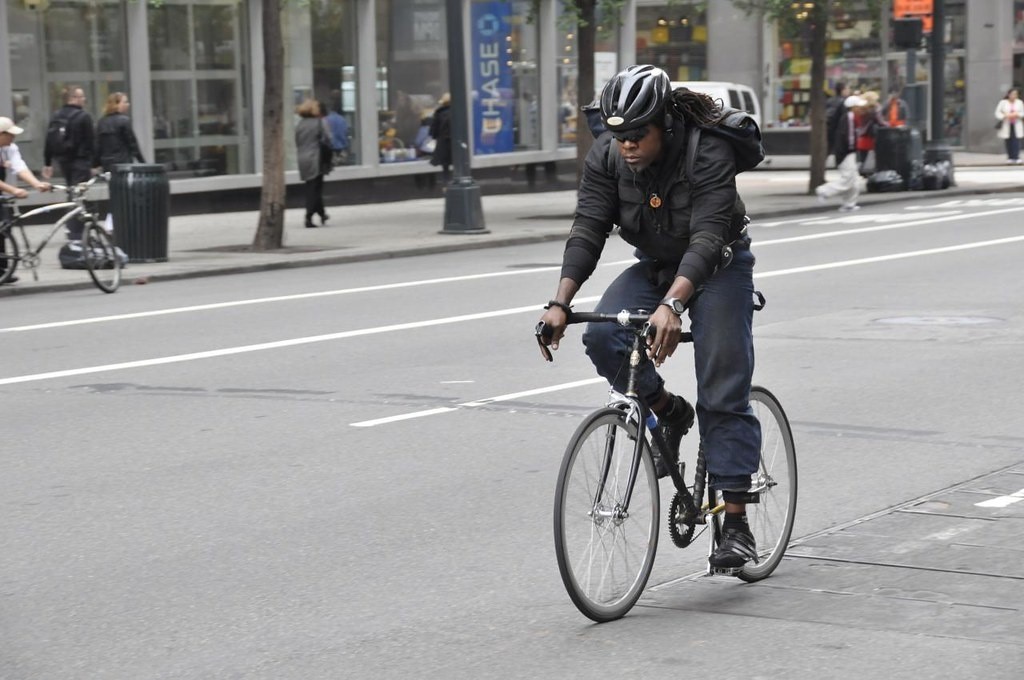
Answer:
<box><xmin>1</xmin><ymin>277</ymin><xmax>18</xmax><ymax>283</ymax></box>
<box><xmin>304</xmin><ymin>217</ymin><xmax>317</xmax><ymax>227</ymax></box>
<box><xmin>1005</xmin><ymin>159</ymin><xmax>1024</xmax><ymax>165</ymax></box>
<box><xmin>321</xmin><ymin>214</ymin><xmax>329</xmax><ymax>225</ymax></box>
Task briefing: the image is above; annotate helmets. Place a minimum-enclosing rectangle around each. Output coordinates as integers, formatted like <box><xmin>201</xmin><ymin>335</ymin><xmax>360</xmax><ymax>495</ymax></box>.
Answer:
<box><xmin>600</xmin><ymin>64</ymin><xmax>672</xmax><ymax>132</ymax></box>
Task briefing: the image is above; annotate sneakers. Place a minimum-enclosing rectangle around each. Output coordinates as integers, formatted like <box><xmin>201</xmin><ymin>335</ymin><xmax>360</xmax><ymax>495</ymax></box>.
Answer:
<box><xmin>648</xmin><ymin>395</ymin><xmax>695</xmax><ymax>479</ymax></box>
<box><xmin>707</xmin><ymin>527</ymin><xmax>760</xmax><ymax>568</ymax></box>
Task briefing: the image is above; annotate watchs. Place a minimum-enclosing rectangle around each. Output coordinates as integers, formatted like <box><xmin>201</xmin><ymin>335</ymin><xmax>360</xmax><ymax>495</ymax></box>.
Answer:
<box><xmin>659</xmin><ymin>297</ymin><xmax>685</xmax><ymax>316</ymax></box>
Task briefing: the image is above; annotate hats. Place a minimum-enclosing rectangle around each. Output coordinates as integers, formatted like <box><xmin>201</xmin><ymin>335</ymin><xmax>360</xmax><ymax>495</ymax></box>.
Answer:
<box><xmin>0</xmin><ymin>116</ymin><xmax>25</xmax><ymax>135</ymax></box>
<box><xmin>843</xmin><ymin>95</ymin><xmax>869</xmax><ymax>109</ymax></box>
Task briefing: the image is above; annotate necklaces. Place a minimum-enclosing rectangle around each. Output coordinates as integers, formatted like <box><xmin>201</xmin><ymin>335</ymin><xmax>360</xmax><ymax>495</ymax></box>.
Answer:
<box><xmin>643</xmin><ymin>150</ymin><xmax>669</xmax><ymax>208</ymax></box>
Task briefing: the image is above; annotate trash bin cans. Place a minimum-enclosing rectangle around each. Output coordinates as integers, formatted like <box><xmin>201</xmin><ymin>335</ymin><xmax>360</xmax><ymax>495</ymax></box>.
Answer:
<box><xmin>108</xmin><ymin>164</ymin><xmax>169</xmax><ymax>263</ymax></box>
<box><xmin>874</xmin><ymin>125</ymin><xmax>923</xmax><ymax>190</ymax></box>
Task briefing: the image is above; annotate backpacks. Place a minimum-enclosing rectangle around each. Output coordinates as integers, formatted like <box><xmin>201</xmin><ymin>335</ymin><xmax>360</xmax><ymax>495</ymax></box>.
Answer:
<box><xmin>606</xmin><ymin>104</ymin><xmax>766</xmax><ymax>189</ymax></box>
<box><xmin>46</xmin><ymin>107</ymin><xmax>84</xmax><ymax>161</ymax></box>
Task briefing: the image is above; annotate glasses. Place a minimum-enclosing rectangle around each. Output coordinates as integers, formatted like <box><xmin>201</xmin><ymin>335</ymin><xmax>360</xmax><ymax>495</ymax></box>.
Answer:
<box><xmin>609</xmin><ymin>121</ymin><xmax>651</xmax><ymax>145</ymax></box>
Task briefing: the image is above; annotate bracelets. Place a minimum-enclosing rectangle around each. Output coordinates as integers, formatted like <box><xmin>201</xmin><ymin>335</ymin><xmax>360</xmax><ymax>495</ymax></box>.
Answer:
<box><xmin>543</xmin><ymin>301</ymin><xmax>572</xmax><ymax>314</ymax></box>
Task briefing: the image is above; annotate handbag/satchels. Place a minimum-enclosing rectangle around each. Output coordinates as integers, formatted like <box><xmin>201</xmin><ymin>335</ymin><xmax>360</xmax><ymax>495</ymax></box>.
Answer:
<box><xmin>313</xmin><ymin>119</ymin><xmax>334</xmax><ymax>175</ymax></box>
<box><xmin>994</xmin><ymin>118</ymin><xmax>1005</xmax><ymax>130</ymax></box>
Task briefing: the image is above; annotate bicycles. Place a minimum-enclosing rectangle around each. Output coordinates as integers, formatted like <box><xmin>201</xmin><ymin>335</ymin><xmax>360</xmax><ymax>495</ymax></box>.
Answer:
<box><xmin>532</xmin><ymin>310</ymin><xmax>800</xmax><ymax>622</ymax></box>
<box><xmin>0</xmin><ymin>171</ymin><xmax>121</xmax><ymax>294</ymax></box>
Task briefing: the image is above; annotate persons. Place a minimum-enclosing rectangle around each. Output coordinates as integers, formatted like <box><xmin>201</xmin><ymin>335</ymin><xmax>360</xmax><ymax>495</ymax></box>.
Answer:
<box><xmin>995</xmin><ymin>89</ymin><xmax>1024</xmax><ymax>164</ymax></box>
<box><xmin>296</xmin><ymin>100</ymin><xmax>332</xmax><ymax>227</ymax></box>
<box><xmin>538</xmin><ymin>64</ymin><xmax>761</xmax><ymax>566</ymax></box>
<box><xmin>561</xmin><ymin>90</ymin><xmax>577</xmax><ymax>129</ymax></box>
<box><xmin>92</xmin><ymin>92</ymin><xmax>146</xmax><ymax>230</ymax></box>
<box><xmin>413</xmin><ymin>113</ymin><xmax>436</xmax><ymax>156</ymax></box>
<box><xmin>0</xmin><ymin>117</ymin><xmax>52</xmax><ymax>283</ymax></box>
<box><xmin>326</xmin><ymin>106</ymin><xmax>348</xmax><ymax>156</ymax></box>
<box><xmin>827</xmin><ymin>82</ymin><xmax>908</xmax><ymax>212</ymax></box>
<box><xmin>430</xmin><ymin>93</ymin><xmax>453</xmax><ymax>180</ymax></box>
<box><xmin>43</xmin><ymin>86</ymin><xmax>94</xmax><ymax>239</ymax></box>
<box><xmin>525</xmin><ymin>89</ymin><xmax>538</xmax><ymax>137</ymax></box>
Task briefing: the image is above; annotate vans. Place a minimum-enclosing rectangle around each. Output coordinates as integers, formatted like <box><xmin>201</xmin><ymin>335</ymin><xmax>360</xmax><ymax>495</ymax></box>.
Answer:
<box><xmin>669</xmin><ymin>80</ymin><xmax>760</xmax><ymax>131</ymax></box>
